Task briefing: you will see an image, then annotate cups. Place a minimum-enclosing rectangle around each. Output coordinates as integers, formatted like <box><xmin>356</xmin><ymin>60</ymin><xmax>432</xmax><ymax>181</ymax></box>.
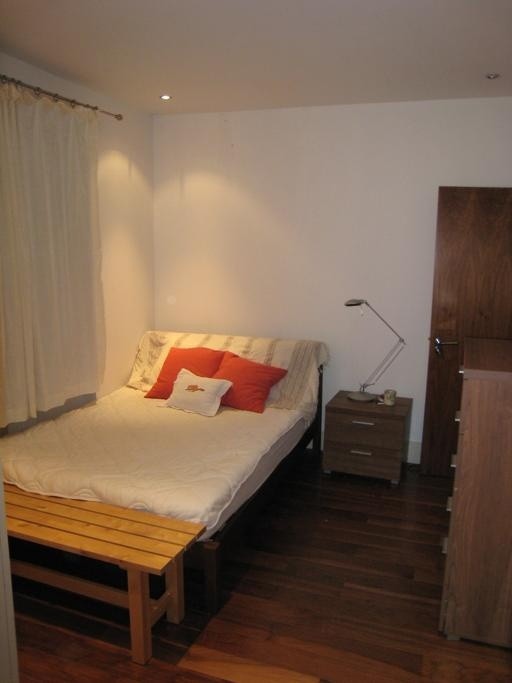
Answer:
<box><xmin>383</xmin><ymin>390</ymin><xmax>397</xmax><ymax>406</ymax></box>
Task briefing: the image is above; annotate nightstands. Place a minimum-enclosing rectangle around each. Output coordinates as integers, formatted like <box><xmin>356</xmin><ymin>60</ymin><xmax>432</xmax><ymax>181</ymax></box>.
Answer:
<box><xmin>325</xmin><ymin>391</ymin><xmax>414</xmax><ymax>487</ymax></box>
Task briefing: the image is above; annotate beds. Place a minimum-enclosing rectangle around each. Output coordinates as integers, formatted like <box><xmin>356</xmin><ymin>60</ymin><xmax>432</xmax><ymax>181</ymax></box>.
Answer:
<box><xmin>0</xmin><ymin>328</ymin><xmax>324</xmax><ymax>601</ymax></box>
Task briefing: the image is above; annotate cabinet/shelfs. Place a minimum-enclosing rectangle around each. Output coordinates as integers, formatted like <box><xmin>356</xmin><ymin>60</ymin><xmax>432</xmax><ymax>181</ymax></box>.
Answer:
<box><xmin>438</xmin><ymin>335</ymin><xmax>511</xmax><ymax>650</ymax></box>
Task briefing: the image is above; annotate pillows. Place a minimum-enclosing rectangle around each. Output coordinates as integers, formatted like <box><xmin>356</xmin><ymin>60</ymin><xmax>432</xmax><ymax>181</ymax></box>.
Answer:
<box><xmin>127</xmin><ymin>330</ymin><xmax>322</xmax><ymax>415</ymax></box>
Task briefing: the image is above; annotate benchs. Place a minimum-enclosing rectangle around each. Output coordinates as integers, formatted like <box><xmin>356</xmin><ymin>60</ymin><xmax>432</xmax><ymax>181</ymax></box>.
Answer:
<box><xmin>3</xmin><ymin>482</ymin><xmax>205</xmax><ymax>666</ymax></box>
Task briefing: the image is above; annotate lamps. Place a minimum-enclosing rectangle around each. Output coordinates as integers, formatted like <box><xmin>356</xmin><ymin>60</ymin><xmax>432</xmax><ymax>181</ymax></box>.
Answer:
<box><xmin>344</xmin><ymin>299</ymin><xmax>407</xmax><ymax>402</ymax></box>
